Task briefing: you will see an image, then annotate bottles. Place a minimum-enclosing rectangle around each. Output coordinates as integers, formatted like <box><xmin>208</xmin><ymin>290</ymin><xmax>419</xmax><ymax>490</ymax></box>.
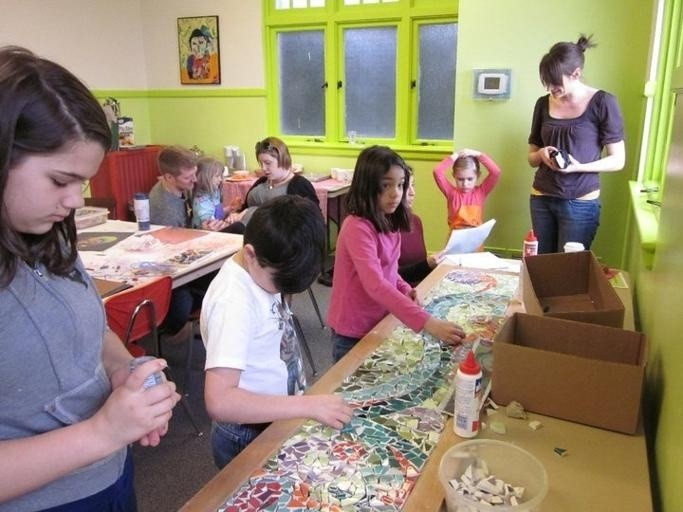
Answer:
<box><xmin>452</xmin><ymin>350</ymin><xmax>485</xmax><ymax>439</ymax></box>
<box><xmin>134</xmin><ymin>192</ymin><xmax>150</xmax><ymax>231</ymax></box>
<box><xmin>224</xmin><ymin>146</ymin><xmax>246</xmax><ymax>171</ymax></box>
<box><xmin>128</xmin><ymin>357</ymin><xmax>162</xmax><ymax>390</ymax></box>
<box><xmin>522</xmin><ymin>229</ymin><xmax>538</xmax><ymax>259</ymax></box>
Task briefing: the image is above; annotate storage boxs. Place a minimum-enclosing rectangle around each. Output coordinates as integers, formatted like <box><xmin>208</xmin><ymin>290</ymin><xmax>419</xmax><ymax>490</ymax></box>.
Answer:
<box><xmin>522</xmin><ymin>250</ymin><xmax>625</xmax><ymax>329</ymax></box>
<box><xmin>491</xmin><ymin>310</ymin><xmax>649</xmax><ymax>435</ymax></box>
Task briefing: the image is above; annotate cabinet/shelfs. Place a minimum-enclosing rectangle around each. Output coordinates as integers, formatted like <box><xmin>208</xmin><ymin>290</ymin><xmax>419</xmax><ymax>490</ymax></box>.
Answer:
<box><xmin>91</xmin><ymin>147</ymin><xmax>162</xmax><ymax>222</ymax></box>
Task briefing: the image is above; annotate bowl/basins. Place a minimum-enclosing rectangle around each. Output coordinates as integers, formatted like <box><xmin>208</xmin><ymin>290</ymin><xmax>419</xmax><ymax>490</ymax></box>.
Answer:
<box><xmin>438</xmin><ymin>440</ymin><xmax>549</xmax><ymax>511</ymax></box>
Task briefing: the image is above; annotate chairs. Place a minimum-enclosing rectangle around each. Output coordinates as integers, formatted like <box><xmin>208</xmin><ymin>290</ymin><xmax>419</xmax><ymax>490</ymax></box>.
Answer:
<box><xmin>102</xmin><ymin>276</ymin><xmax>204</xmax><ymax>437</ymax></box>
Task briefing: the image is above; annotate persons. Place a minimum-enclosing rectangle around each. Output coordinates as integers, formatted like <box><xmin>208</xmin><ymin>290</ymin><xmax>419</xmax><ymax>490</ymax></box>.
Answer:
<box><xmin>198</xmin><ymin>195</ymin><xmax>355</xmax><ymax>471</ymax></box>
<box><xmin>527</xmin><ymin>31</ymin><xmax>626</xmax><ymax>255</ymax></box>
<box><xmin>189</xmin><ymin>137</ymin><xmax>321</xmax><ymax>296</ymax></box>
<box><xmin>394</xmin><ymin>166</ymin><xmax>447</xmax><ymax>289</ymax></box>
<box><xmin>432</xmin><ymin>149</ymin><xmax>502</xmax><ymax>254</ymax></box>
<box><xmin>0</xmin><ymin>43</ymin><xmax>182</xmax><ymax>510</ymax></box>
<box><xmin>147</xmin><ymin>143</ymin><xmax>229</xmax><ymax>232</ymax></box>
<box><xmin>325</xmin><ymin>144</ymin><xmax>467</xmax><ymax>366</ymax></box>
<box><xmin>191</xmin><ymin>154</ymin><xmax>244</xmax><ymax>232</ymax></box>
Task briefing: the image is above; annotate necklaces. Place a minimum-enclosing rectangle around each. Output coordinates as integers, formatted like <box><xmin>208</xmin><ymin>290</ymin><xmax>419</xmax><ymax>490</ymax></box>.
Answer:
<box><xmin>268</xmin><ymin>172</ymin><xmax>291</xmax><ymax>190</ymax></box>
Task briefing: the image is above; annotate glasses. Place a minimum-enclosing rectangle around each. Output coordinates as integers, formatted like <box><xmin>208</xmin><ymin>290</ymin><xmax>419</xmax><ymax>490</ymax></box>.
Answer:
<box><xmin>256</xmin><ymin>141</ymin><xmax>276</xmax><ymax>151</ymax></box>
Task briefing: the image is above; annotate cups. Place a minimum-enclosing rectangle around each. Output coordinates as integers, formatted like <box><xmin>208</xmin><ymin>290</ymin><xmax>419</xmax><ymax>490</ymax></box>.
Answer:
<box><xmin>563</xmin><ymin>241</ymin><xmax>586</xmax><ymax>253</ymax></box>
<box><xmin>329</xmin><ymin>168</ymin><xmax>353</xmax><ymax>181</ymax></box>
<box><xmin>348</xmin><ymin>130</ymin><xmax>356</xmax><ymax>144</ymax></box>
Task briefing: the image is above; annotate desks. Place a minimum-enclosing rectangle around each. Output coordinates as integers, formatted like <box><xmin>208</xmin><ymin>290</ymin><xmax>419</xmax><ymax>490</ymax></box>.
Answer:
<box><xmin>177</xmin><ymin>257</ymin><xmax>656</xmax><ymax>512</ymax></box>
<box><xmin>222</xmin><ymin>174</ymin><xmax>352</xmax><ymax>275</ymax></box>
<box><xmin>76</xmin><ymin>219</ymin><xmax>244</xmax><ymax>304</ymax></box>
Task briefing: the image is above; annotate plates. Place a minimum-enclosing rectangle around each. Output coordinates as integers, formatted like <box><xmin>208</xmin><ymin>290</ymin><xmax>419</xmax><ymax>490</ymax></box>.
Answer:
<box><xmin>226</xmin><ymin>177</ymin><xmax>250</xmax><ymax>182</ymax></box>
